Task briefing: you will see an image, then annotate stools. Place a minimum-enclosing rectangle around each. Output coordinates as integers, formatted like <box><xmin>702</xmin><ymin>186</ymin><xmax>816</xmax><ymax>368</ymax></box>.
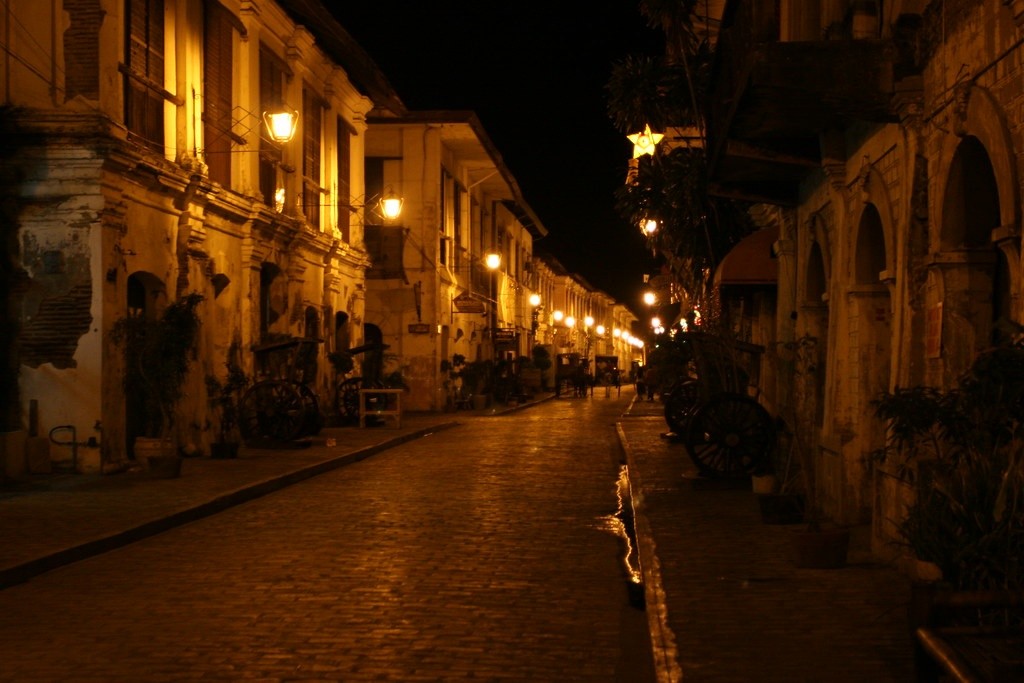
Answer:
<box><xmin>916</xmin><ymin>624</ymin><xmax>1024</xmax><ymax>683</ymax></box>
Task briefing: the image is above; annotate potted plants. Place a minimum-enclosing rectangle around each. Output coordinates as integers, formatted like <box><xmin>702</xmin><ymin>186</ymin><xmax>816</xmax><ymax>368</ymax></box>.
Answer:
<box><xmin>866</xmin><ymin>345</ymin><xmax>1024</xmax><ymax>640</ymax></box>
<box><xmin>746</xmin><ymin>416</ymin><xmax>784</xmax><ymax>495</ymax></box>
<box><xmin>205</xmin><ymin>367</ymin><xmax>251</xmax><ymax>459</ymax></box>
<box><xmin>111</xmin><ymin>292</ymin><xmax>205</xmax><ymax>459</ymax></box>
<box><xmin>458</xmin><ymin>360</ymin><xmax>494</xmax><ymax>411</ymax></box>
<box><xmin>764</xmin><ymin>335</ymin><xmax>849</xmax><ymax>569</ymax></box>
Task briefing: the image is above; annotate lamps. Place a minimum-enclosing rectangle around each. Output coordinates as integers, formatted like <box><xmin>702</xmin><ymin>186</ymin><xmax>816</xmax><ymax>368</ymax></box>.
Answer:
<box><xmin>199</xmin><ymin>97</ymin><xmax>300</xmax><ymax>158</ymax></box>
<box><xmin>457</xmin><ymin>247</ymin><xmax>503</xmax><ymax>272</ymax></box>
<box><xmin>341</xmin><ymin>185</ymin><xmax>405</xmax><ymax>221</ymax></box>
